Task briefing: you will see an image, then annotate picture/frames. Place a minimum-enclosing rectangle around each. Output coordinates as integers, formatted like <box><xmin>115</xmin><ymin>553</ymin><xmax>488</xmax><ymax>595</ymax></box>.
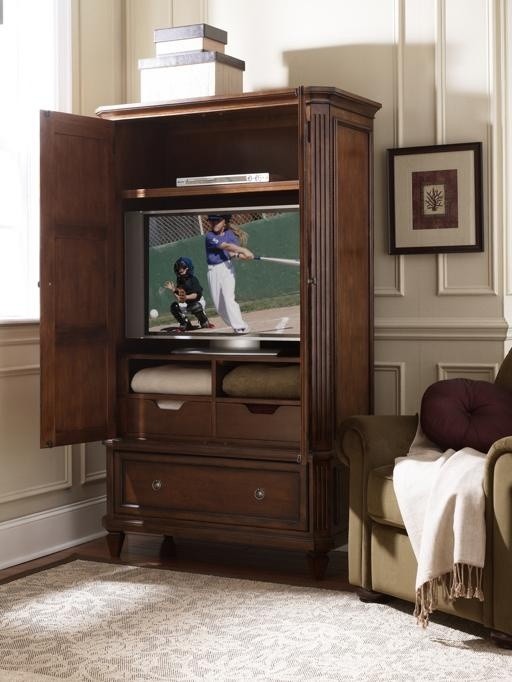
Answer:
<box><xmin>385</xmin><ymin>141</ymin><xmax>485</xmax><ymax>256</ymax></box>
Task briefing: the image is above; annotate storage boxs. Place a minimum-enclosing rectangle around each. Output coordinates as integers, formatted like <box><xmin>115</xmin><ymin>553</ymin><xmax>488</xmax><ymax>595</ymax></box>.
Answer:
<box><xmin>137</xmin><ymin>24</ymin><xmax>245</xmax><ymax>104</ymax></box>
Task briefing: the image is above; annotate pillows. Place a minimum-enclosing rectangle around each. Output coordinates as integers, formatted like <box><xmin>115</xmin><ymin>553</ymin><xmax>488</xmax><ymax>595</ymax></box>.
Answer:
<box><xmin>420</xmin><ymin>377</ymin><xmax>509</xmax><ymax>454</ymax></box>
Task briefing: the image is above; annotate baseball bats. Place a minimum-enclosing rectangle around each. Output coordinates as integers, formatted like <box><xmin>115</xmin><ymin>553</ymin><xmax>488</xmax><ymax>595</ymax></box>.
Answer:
<box><xmin>234</xmin><ymin>252</ymin><xmax>300</xmax><ymax>265</ymax></box>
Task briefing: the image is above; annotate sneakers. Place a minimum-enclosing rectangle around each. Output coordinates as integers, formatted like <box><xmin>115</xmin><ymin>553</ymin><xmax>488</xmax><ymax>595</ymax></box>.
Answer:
<box><xmin>201</xmin><ymin>321</ymin><xmax>215</xmax><ymax>328</ymax></box>
<box><xmin>233</xmin><ymin>324</ymin><xmax>250</xmax><ymax>335</ymax></box>
<box><xmin>176</xmin><ymin>322</ymin><xmax>191</xmax><ymax>334</ymax></box>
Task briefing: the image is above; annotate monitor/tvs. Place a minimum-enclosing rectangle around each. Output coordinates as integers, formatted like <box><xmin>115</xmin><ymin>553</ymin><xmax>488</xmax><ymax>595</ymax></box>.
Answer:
<box><xmin>125</xmin><ymin>204</ymin><xmax>300</xmax><ymax>356</ymax></box>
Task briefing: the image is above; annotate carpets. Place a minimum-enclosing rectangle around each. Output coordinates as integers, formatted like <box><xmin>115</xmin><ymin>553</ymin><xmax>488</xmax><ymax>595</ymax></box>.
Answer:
<box><xmin>2</xmin><ymin>554</ymin><xmax>512</xmax><ymax>681</ymax></box>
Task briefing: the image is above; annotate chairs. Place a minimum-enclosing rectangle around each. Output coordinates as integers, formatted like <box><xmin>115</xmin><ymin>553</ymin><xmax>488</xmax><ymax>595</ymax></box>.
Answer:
<box><xmin>335</xmin><ymin>347</ymin><xmax>510</xmax><ymax>650</ymax></box>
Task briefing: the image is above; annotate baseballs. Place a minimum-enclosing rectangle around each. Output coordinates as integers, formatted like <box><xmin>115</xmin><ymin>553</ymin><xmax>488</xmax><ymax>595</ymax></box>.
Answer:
<box><xmin>150</xmin><ymin>309</ymin><xmax>158</xmax><ymax>319</ymax></box>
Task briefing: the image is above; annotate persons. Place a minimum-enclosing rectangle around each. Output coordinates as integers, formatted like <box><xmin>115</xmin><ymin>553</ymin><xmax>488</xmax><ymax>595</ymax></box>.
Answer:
<box><xmin>204</xmin><ymin>214</ymin><xmax>255</xmax><ymax>335</ymax></box>
<box><xmin>164</xmin><ymin>256</ymin><xmax>209</xmax><ymax>330</ymax></box>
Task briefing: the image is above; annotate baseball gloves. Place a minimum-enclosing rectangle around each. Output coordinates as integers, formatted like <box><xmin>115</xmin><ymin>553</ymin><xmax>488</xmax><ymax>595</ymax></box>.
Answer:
<box><xmin>174</xmin><ymin>288</ymin><xmax>186</xmax><ymax>303</ymax></box>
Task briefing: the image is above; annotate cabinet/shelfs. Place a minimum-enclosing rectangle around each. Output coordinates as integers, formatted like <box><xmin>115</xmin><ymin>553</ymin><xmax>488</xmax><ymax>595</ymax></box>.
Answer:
<box><xmin>39</xmin><ymin>85</ymin><xmax>383</xmax><ymax>580</ymax></box>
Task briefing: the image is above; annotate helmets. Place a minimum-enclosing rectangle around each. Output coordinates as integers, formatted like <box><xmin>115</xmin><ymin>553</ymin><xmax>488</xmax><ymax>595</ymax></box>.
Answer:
<box><xmin>174</xmin><ymin>257</ymin><xmax>193</xmax><ymax>279</ymax></box>
<box><xmin>206</xmin><ymin>212</ymin><xmax>232</xmax><ymax>221</ymax></box>
